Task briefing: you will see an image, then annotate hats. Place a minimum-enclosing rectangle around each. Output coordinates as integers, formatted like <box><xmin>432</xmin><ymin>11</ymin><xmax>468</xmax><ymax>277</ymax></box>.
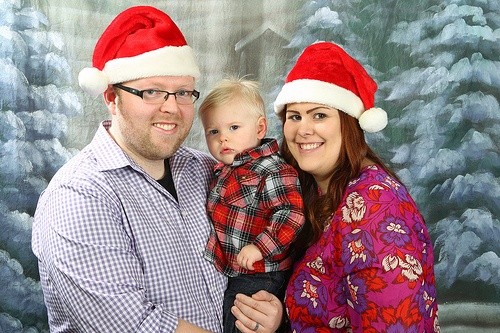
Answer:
<box><xmin>272</xmin><ymin>41</ymin><xmax>394</xmax><ymax>136</ymax></box>
<box><xmin>79</xmin><ymin>5</ymin><xmax>201</xmax><ymax>96</ymax></box>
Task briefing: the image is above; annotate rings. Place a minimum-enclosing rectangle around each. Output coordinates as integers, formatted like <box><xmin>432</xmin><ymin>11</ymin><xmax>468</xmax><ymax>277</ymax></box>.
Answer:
<box><xmin>254</xmin><ymin>322</ymin><xmax>259</xmax><ymax>331</ymax></box>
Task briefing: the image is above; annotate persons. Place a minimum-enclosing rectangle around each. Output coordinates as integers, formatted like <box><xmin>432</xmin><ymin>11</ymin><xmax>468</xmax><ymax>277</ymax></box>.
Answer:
<box><xmin>198</xmin><ymin>75</ymin><xmax>305</xmax><ymax>333</ymax></box>
<box><xmin>30</xmin><ymin>6</ymin><xmax>286</xmax><ymax>333</ymax></box>
<box><xmin>273</xmin><ymin>42</ymin><xmax>441</xmax><ymax>333</ymax></box>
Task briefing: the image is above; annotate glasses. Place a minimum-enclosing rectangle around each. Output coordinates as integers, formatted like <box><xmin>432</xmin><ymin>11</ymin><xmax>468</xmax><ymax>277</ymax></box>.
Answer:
<box><xmin>112</xmin><ymin>84</ymin><xmax>201</xmax><ymax>107</ymax></box>
